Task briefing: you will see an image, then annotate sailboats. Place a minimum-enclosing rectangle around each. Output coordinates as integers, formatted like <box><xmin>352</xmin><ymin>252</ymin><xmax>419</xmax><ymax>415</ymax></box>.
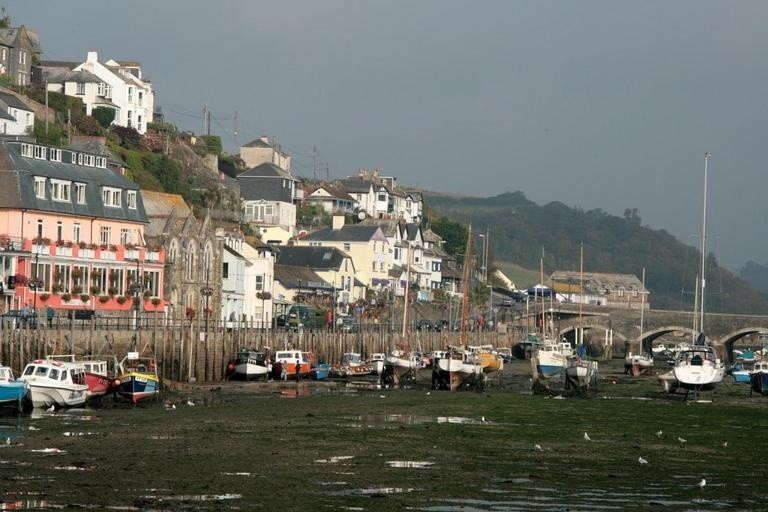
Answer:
<box><xmin>523</xmin><ymin>241</ymin><xmax>598</xmax><ymax>399</ymax></box>
<box><xmin>227</xmin><ymin>220</ymin><xmax>513</xmax><ymax>393</ymax></box>
<box><xmin>624</xmin><ymin>152</ymin><xmax>768</xmax><ymax>402</ymax></box>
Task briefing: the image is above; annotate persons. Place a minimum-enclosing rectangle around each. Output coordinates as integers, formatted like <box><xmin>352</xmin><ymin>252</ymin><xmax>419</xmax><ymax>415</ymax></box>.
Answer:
<box><xmin>266</xmin><ymin>359</ymin><xmax>274</xmax><ymax>382</ymax></box>
<box><xmin>22</xmin><ymin>303</ymin><xmax>30</xmax><ymax>322</ymax></box>
<box><xmin>295</xmin><ymin>359</ymin><xmax>302</xmax><ymax>383</ymax></box>
<box><xmin>281</xmin><ymin>360</ymin><xmax>289</xmax><ymax>381</ymax></box>
<box><xmin>47</xmin><ymin>304</ymin><xmax>55</xmax><ymax>328</ymax></box>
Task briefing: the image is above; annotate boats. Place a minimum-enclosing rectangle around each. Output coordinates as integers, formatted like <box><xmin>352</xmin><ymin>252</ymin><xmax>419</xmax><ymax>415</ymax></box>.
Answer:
<box><xmin>0</xmin><ymin>348</ymin><xmax>160</xmax><ymax>411</ymax></box>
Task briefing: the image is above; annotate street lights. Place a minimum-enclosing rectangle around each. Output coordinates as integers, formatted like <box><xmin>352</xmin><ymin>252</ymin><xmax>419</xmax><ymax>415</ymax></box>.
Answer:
<box><xmin>29</xmin><ymin>253</ymin><xmax>44</xmax><ymax>329</ymax></box>
<box><xmin>124</xmin><ymin>258</ymin><xmax>143</xmax><ymax>330</ymax></box>
<box><xmin>200</xmin><ymin>269</ymin><xmax>213</xmax><ymax>332</ymax></box>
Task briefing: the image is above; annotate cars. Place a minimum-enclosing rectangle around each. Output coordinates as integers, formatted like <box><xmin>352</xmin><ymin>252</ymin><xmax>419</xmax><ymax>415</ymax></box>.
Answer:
<box><xmin>0</xmin><ymin>310</ymin><xmax>38</xmax><ymax>329</ymax></box>
